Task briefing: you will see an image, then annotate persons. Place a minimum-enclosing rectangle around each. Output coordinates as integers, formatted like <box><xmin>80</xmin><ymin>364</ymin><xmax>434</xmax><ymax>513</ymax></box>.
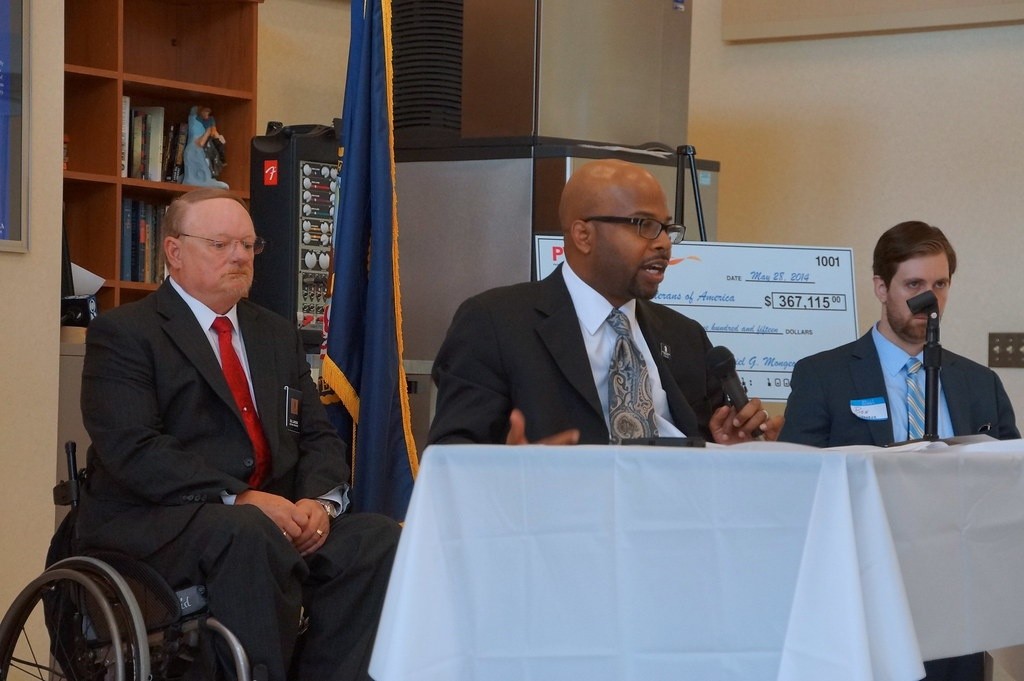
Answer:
<box><xmin>778</xmin><ymin>220</ymin><xmax>1021</xmax><ymax>680</ymax></box>
<box><xmin>182</xmin><ymin>104</ymin><xmax>230</xmax><ymax>190</ymax></box>
<box><xmin>73</xmin><ymin>191</ymin><xmax>402</xmax><ymax>680</ymax></box>
<box><xmin>423</xmin><ymin>156</ymin><xmax>784</xmax><ymax>444</ymax></box>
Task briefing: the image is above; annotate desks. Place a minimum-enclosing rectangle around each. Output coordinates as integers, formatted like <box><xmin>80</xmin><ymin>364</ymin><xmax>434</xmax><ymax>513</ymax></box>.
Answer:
<box><xmin>367</xmin><ymin>438</ymin><xmax>1024</xmax><ymax>681</ymax></box>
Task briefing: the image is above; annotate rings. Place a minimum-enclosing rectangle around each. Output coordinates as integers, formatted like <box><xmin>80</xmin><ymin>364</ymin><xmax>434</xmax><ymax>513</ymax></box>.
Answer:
<box><xmin>283</xmin><ymin>532</ymin><xmax>286</xmax><ymax>536</ymax></box>
<box><xmin>762</xmin><ymin>410</ymin><xmax>769</xmax><ymax>419</ymax></box>
<box><xmin>317</xmin><ymin>530</ymin><xmax>323</xmax><ymax>535</ymax></box>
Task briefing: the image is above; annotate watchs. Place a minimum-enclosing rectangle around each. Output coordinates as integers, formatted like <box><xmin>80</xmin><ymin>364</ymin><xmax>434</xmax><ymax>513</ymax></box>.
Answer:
<box><xmin>316</xmin><ymin>499</ymin><xmax>337</xmax><ymax>517</ymax></box>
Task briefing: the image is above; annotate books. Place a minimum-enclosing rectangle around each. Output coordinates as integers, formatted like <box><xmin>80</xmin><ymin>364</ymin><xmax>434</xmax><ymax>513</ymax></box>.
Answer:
<box><xmin>63</xmin><ymin>132</ymin><xmax>68</xmax><ymax>170</ymax></box>
<box><xmin>121</xmin><ymin>96</ymin><xmax>187</xmax><ymax>182</ymax></box>
<box><xmin>122</xmin><ymin>199</ymin><xmax>170</xmax><ymax>283</ymax></box>
<box><xmin>61</xmin><ymin>199</ymin><xmax>74</xmax><ymax>296</ymax></box>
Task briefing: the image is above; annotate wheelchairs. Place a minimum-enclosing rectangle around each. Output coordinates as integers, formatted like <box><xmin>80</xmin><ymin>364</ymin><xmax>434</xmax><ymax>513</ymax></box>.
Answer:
<box><xmin>0</xmin><ymin>441</ymin><xmax>310</xmax><ymax>681</ymax></box>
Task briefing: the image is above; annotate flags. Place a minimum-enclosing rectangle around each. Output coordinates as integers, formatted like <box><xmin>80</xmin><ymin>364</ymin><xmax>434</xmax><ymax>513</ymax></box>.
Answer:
<box><xmin>315</xmin><ymin>0</ymin><xmax>419</xmax><ymax>525</ymax></box>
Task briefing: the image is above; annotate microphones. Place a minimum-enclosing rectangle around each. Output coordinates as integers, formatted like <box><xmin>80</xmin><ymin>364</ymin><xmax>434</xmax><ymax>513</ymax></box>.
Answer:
<box><xmin>706</xmin><ymin>345</ymin><xmax>769</xmax><ymax>441</ymax></box>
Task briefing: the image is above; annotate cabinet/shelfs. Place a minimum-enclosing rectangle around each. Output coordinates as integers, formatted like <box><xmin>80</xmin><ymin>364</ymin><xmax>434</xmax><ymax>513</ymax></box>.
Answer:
<box><xmin>56</xmin><ymin>1</ymin><xmax>259</xmax><ymax>537</ymax></box>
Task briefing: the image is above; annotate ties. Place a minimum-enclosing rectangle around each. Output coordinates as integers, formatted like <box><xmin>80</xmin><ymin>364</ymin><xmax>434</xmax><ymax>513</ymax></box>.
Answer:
<box><xmin>211</xmin><ymin>316</ymin><xmax>269</xmax><ymax>491</ymax></box>
<box><xmin>905</xmin><ymin>358</ymin><xmax>926</xmax><ymax>440</ymax></box>
<box><xmin>606</xmin><ymin>309</ymin><xmax>658</xmax><ymax>445</ymax></box>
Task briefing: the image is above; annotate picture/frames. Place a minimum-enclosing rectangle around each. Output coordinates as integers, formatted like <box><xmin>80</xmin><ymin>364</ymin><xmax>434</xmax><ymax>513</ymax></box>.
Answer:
<box><xmin>0</xmin><ymin>0</ymin><xmax>33</xmax><ymax>253</ymax></box>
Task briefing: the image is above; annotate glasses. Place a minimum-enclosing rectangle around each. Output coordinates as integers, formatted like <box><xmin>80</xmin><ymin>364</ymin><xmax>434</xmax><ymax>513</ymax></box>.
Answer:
<box><xmin>581</xmin><ymin>216</ymin><xmax>686</xmax><ymax>245</ymax></box>
<box><xmin>176</xmin><ymin>233</ymin><xmax>266</xmax><ymax>255</ymax></box>
